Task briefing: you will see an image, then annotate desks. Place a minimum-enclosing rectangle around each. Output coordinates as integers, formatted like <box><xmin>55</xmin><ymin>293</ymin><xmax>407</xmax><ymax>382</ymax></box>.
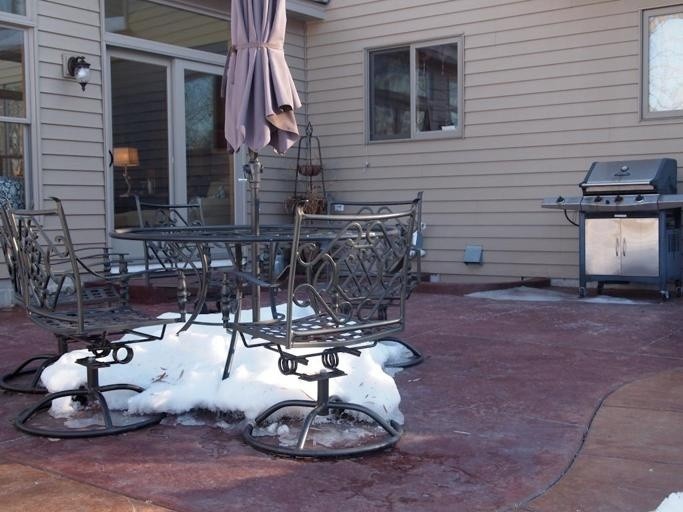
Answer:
<box><xmin>108</xmin><ymin>225</ymin><xmax>396</xmax><ymax>381</ymax></box>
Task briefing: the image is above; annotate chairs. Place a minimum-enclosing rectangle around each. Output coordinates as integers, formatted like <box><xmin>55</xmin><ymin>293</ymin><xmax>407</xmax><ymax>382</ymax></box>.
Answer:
<box><xmin>133</xmin><ymin>194</ymin><xmax>245</xmax><ymax>316</ymax></box>
<box><xmin>222</xmin><ymin>197</ymin><xmax>421</xmax><ymax>457</ymax></box>
<box><xmin>305</xmin><ymin>189</ymin><xmax>424</xmax><ymax>370</ymax></box>
<box><xmin>0</xmin><ymin>201</ymin><xmax>133</xmax><ymax>393</ymax></box>
<box><xmin>0</xmin><ymin>196</ymin><xmax>186</xmax><ymax>438</ymax></box>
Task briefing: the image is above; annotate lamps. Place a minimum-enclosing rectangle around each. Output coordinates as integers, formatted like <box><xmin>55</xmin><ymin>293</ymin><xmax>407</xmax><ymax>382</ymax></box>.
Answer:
<box><xmin>68</xmin><ymin>55</ymin><xmax>92</xmax><ymax>92</ymax></box>
<box><xmin>112</xmin><ymin>146</ymin><xmax>140</xmax><ymax>200</ymax></box>
